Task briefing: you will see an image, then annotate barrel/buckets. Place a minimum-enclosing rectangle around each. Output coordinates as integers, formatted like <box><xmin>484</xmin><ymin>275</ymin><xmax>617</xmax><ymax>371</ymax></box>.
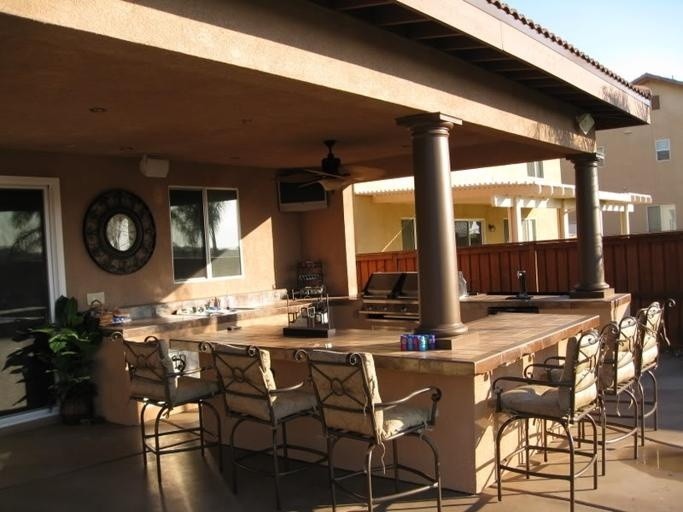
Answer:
<box><xmin>458</xmin><ymin>271</ymin><xmax>467</xmax><ymax>300</ymax></box>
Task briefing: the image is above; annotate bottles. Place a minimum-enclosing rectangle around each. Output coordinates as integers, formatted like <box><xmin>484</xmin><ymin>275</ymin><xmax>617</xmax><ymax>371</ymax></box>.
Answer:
<box><xmin>400</xmin><ymin>334</ymin><xmax>437</xmax><ymax>352</ymax></box>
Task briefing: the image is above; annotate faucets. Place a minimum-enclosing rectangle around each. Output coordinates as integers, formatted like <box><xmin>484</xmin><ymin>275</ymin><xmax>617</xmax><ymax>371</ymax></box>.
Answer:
<box><xmin>214</xmin><ymin>297</ymin><xmax>221</xmax><ymax>308</ymax></box>
<box><xmin>516</xmin><ymin>271</ymin><xmax>523</xmax><ymax>279</ymax></box>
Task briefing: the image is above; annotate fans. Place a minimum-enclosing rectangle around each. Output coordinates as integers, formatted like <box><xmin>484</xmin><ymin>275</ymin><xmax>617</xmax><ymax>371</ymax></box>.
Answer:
<box><xmin>269</xmin><ymin>135</ymin><xmax>385</xmax><ymax>192</ymax></box>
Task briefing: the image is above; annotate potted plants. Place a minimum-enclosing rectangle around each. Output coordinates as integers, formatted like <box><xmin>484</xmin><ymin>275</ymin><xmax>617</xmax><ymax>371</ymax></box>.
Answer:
<box><xmin>1</xmin><ymin>290</ymin><xmax>117</xmax><ymax>433</ymax></box>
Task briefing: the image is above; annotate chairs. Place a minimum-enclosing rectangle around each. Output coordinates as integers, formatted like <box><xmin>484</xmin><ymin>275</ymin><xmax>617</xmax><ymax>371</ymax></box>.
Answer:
<box><xmin>197</xmin><ymin>340</ymin><xmax>328</xmax><ymax>512</ymax></box>
<box><xmin>294</xmin><ymin>345</ymin><xmax>445</xmax><ymax>511</ymax></box>
<box><xmin>490</xmin><ymin>322</ymin><xmax>612</xmax><ymax>512</ymax></box>
<box><xmin>581</xmin><ymin>298</ymin><xmax>676</xmax><ymax>446</ymax></box>
<box><xmin>541</xmin><ymin>313</ymin><xmax>642</xmax><ymax>476</ymax></box>
<box><xmin>111</xmin><ymin>329</ymin><xmax>224</xmax><ymax>484</ymax></box>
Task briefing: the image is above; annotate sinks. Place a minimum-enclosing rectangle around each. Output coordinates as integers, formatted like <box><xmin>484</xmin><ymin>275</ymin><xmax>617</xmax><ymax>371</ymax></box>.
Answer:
<box><xmin>187</xmin><ymin>309</ymin><xmax>241</xmax><ymax>315</ymax></box>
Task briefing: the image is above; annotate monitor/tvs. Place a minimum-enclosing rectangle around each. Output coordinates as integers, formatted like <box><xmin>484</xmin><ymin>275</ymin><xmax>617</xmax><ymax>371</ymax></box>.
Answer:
<box><xmin>278</xmin><ymin>176</ymin><xmax>328</xmax><ymax>213</ymax></box>
